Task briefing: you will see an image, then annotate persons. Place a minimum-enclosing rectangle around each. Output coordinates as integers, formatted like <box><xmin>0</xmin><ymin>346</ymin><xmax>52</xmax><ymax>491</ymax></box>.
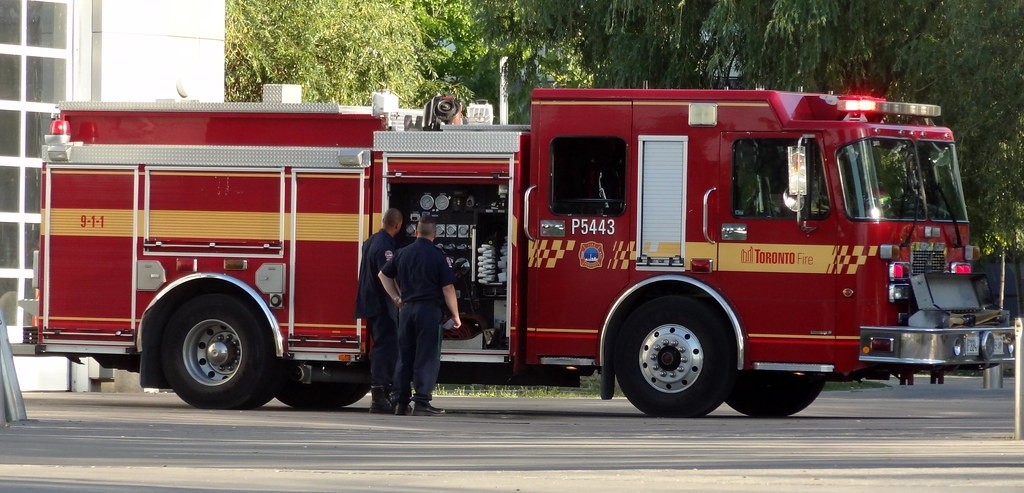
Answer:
<box><xmin>354</xmin><ymin>208</ymin><xmax>412</xmax><ymax>414</ymax></box>
<box><xmin>377</xmin><ymin>215</ymin><xmax>461</xmax><ymax>416</ymax></box>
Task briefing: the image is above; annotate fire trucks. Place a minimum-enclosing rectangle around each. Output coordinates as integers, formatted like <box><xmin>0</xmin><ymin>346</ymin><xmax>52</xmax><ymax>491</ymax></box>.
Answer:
<box><xmin>17</xmin><ymin>57</ymin><xmax>1015</xmax><ymax>418</ymax></box>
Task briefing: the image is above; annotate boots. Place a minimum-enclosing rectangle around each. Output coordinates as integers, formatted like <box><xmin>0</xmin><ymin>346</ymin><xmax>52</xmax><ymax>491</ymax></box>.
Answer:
<box><xmin>369</xmin><ymin>384</ymin><xmax>396</xmax><ymax>414</ymax></box>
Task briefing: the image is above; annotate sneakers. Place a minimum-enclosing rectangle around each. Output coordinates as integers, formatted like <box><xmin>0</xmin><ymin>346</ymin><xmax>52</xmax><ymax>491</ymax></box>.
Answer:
<box><xmin>413</xmin><ymin>400</ymin><xmax>446</xmax><ymax>416</ymax></box>
<box><xmin>394</xmin><ymin>401</ymin><xmax>413</xmax><ymax>415</ymax></box>
